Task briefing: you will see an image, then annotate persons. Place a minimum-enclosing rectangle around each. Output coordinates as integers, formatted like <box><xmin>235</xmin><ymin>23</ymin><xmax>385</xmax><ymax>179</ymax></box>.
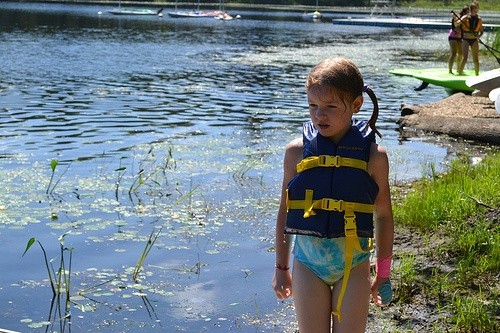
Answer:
<box><xmin>456</xmin><ymin>0</ymin><xmax>483</xmax><ymax>76</ymax></box>
<box><xmin>271</xmin><ymin>56</ymin><xmax>395</xmax><ymax>332</ymax></box>
<box><xmin>447</xmin><ymin>6</ymin><xmax>469</xmax><ymax>74</ymax></box>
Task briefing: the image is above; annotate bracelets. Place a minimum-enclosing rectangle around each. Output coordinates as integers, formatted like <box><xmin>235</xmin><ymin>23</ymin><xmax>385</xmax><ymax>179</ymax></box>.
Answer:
<box><xmin>275</xmin><ymin>264</ymin><xmax>290</xmax><ymax>271</ymax></box>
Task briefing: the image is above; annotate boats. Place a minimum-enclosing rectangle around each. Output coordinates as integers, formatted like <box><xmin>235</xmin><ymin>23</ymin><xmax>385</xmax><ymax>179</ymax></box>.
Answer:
<box><xmin>388</xmin><ymin>66</ymin><xmax>484</xmax><ymax>90</ymax></box>
<box><xmin>304</xmin><ymin>11</ymin><xmax>322</xmax><ymax>18</ymax></box>
<box><xmin>107</xmin><ymin>8</ymin><xmax>164</xmax><ymax>15</ymax></box>
<box><xmin>215</xmin><ymin>15</ymin><xmax>233</xmax><ymax>20</ymax></box>
<box><xmin>168</xmin><ymin>11</ymin><xmax>228</xmax><ymax>17</ymax></box>
